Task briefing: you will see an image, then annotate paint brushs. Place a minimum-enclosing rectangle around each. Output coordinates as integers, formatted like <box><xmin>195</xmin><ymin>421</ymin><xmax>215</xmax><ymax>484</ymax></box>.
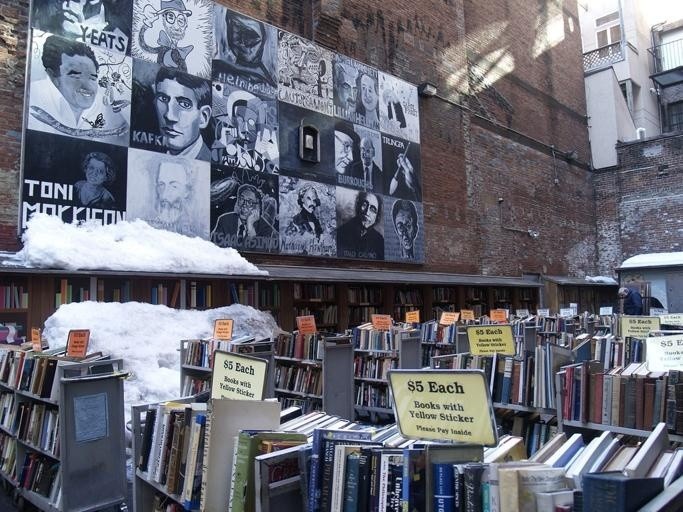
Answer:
<box><xmin>389</xmin><ymin>142</ymin><xmax>413</xmax><ymax>197</ymax></box>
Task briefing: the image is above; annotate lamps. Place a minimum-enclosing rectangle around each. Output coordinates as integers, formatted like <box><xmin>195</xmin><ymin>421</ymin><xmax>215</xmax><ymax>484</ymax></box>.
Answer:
<box><xmin>418</xmin><ymin>79</ymin><xmax>440</xmax><ymax>100</ymax></box>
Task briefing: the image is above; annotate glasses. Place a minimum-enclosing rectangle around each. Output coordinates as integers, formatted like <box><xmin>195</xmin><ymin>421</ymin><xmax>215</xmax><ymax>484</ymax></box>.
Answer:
<box><xmin>335</xmin><ymin>135</ymin><xmax>352</xmax><ymax>153</ymax></box>
<box><xmin>238</xmin><ymin>198</ymin><xmax>258</xmax><ymax>208</ymax></box>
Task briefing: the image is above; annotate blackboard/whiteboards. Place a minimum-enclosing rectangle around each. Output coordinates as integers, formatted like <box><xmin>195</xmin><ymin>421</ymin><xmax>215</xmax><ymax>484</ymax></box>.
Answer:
<box><xmin>60</xmin><ymin>371</ymin><xmax>126</xmax><ymax>511</ymax></box>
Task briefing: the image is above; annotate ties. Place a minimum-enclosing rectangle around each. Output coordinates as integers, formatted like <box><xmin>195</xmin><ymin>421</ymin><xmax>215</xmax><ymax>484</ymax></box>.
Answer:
<box><xmin>238</xmin><ymin>224</ymin><xmax>245</xmax><ymax>244</ymax></box>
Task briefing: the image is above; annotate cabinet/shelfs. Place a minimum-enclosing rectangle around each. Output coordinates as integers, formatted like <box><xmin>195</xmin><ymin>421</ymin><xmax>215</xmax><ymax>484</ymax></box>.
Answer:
<box><xmin>2</xmin><ymin>264</ymin><xmax>680</xmax><ymax>509</ymax></box>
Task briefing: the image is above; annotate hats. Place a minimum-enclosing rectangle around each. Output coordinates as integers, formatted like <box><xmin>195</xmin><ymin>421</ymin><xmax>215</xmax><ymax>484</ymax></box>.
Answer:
<box><xmin>335</xmin><ymin>120</ymin><xmax>360</xmax><ymax>144</ymax></box>
